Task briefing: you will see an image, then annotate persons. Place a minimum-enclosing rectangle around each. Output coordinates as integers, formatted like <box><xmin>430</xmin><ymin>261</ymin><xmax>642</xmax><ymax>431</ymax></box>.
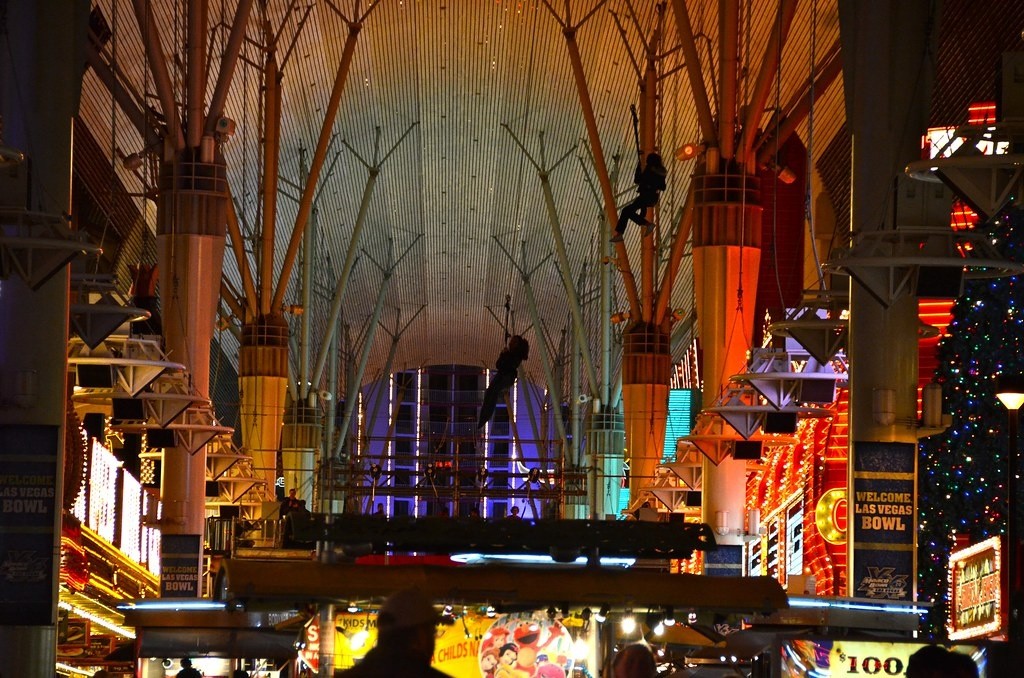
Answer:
<box><xmin>233</xmin><ymin>670</ymin><xmax>248</xmax><ymax>678</ymax></box>
<box><xmin>464</xmin><ymin>507</ymin><xmax>483</xmax><ymax>523</ymax></box>
<box><xmin>281</xmin><ymin>489</ymin><xmax>311</xmax><ymax>520</ymax></box>
<box><xmin>176</xmin><ymin>658</ymin><xmax>202</xmax><ymax>678</ymax></box>
<box><xmin>614</xmin><ymin>644</ymin><xmax>656</xmax><ymax>678</ymax></box>
<box><xmin>373</xmin><ymin>503</ymin><xmax>387</xmax><ymax>555</ymax></box>
<box><xmin>609</xmin><ymin>153</ymin><xmax>667</xmax><ymax>242</ymax></box>
<box><xmin>906</xmin><ymin>645</ymin><xmax>980</xmax><ymax>678</ymax></box>
<box><xmin>506</xmin><ymin>506</ymin><xmax>523</xmax><ymax>523</ymax></box>
<box><xmin>334</xmin><ymin>591</ymin><xmax>455</xmax><ymax>678</ymax></box>
<box><xmin>478</xmin><ymin>334</ymin><xmax>529</xmax><ymax>429</ymax></box>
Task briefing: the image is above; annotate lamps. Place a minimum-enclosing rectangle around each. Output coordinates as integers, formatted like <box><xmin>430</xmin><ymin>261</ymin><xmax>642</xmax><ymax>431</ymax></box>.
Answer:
<box><xmin>595</xmin><ymin>603</ymin><xmax>611</xmax><ymax>621</ymax></box>
<box><xmin>664</xmin><ymin>606</ymin><xmax>675</xmax><ymax>626</ymax></box>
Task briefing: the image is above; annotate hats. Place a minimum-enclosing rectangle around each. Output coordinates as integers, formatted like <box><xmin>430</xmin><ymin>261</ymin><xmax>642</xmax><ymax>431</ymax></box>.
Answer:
<box><xmin>375</xmin><ymin>589</ymin><xmax>453</xmax><ymax>633</ymax></box>
